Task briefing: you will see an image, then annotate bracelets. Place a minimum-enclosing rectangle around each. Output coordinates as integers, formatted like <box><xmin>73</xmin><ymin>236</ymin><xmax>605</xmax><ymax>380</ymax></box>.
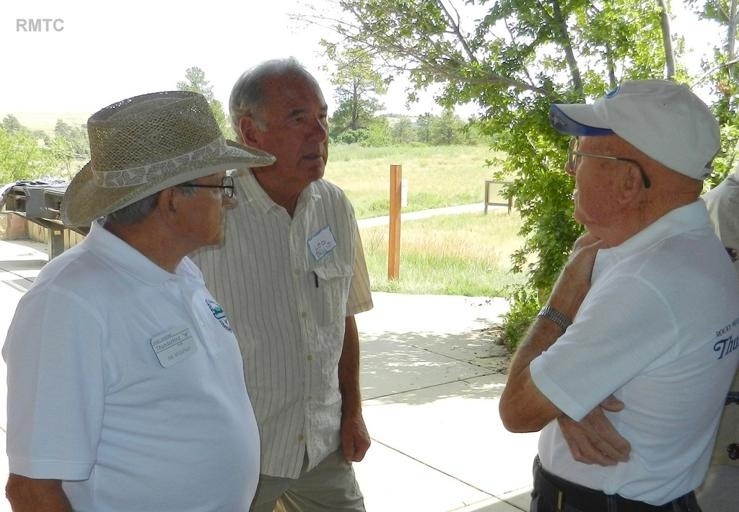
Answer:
<box><xmin>537</xmin><ymin>305</ymin><xmax>572</xmax><ymax>330</ymax></box>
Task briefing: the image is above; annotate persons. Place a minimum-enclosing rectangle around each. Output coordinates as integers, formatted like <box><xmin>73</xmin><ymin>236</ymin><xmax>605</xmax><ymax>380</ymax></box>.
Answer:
<box><xmin>499</xmin><ymin>76</ymin><xmax>738</xmax><ymax>511</ymax></box>
<box><xmin>179</xmin><ymin>55</ymin><xmax>373</xmax><ymax>511</ymax></box>
<box><xmin>1</xmin><ymin>92</ymin><xmax>261</xmax><ymax>511</ymax></box>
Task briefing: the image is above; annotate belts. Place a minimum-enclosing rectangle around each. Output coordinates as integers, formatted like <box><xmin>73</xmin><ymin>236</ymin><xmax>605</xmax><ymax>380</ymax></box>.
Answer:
<box><xmin>532</xmin><ymin>454</ymin><xmax>687</xmax><ymax>512</ymax></box>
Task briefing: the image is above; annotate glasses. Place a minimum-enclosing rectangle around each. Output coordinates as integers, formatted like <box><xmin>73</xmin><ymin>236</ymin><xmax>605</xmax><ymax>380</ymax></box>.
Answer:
<box><xmin>181</xmin><ymin>176</ymin><xmax>234</xmax><ymax>199</ymax></box>
<box><xmin>568</xmin><ymin>138</ymin><xmax>652</xmax><ymax>188</ymax></box>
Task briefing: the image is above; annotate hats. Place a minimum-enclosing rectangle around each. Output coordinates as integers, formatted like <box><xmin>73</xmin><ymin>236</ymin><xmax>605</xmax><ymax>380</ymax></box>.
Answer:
<box><xmin>59</xmin><ymin>91</ymin><xmax>277</xmax><ymax>228</ymax></box>
<box><xmin>550</xmin><ymin>79</ymin><xmax>721</xmax><ymax>181</ymax></box>
